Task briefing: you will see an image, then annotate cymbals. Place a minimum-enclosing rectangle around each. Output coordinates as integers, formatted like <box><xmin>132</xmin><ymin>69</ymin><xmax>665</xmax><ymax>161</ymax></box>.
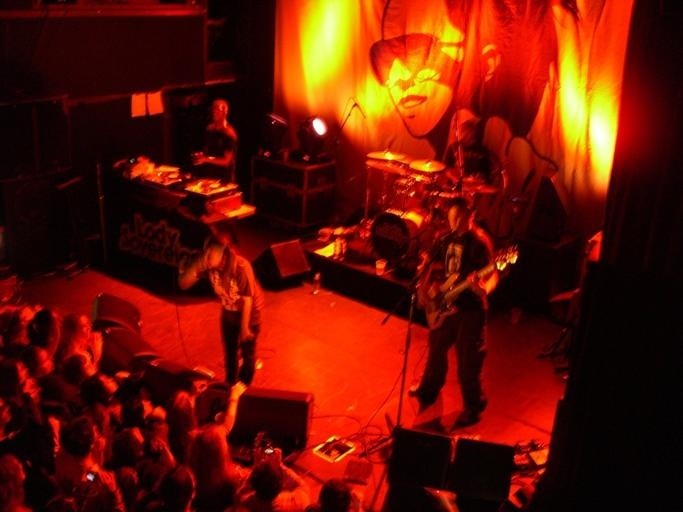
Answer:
<box><xmin>410</xmin><ymin>159</ymin><xmax>446</xmax><ymax>171</ymax></box>
<box><xmin>366</xmin><ymin>152</ymin><xmax>406</xmax><ymax>160</ymax></box>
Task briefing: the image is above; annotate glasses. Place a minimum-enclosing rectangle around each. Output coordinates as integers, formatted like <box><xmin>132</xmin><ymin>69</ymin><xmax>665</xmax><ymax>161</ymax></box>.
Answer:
<box><xmin>369</xmin><ymin>33</ymin><xmax>464</xmax><ymax>85</ymax></box>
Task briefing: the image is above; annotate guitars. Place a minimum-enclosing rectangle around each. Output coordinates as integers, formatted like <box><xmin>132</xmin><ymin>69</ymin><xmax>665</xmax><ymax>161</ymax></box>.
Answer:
<box><xmin>424</xmin><ymin>246</ymin><xmax>519</xmax><ymax>333</ymax></box>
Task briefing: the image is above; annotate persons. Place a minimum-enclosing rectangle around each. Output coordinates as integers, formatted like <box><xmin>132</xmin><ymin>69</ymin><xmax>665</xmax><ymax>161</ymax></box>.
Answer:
<box><xmin>420</xmin><ymin>117</ymin><xmax>496</xmax><ymax>234</ymax></box>
<box><xmin>185</xmin><ymin>96</ymin><xmax>239</xmax><ymax>184</ymax></box>
<box><xmin>406</xmin><ymin>194</ymin><xmax>491</xmax><ymax>432</ymax></box>
<box><xmin>173</xmin><ymin>231</ymin><xmax>265</xmax><ymax>389</ymax></box>
<box><xmin>1</xmin><ymin>301</ymin><xmax>354</xmax><ymax>511</ymax></box>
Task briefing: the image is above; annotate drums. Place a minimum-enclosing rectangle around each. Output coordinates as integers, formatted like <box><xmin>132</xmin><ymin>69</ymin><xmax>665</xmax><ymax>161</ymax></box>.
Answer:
<box><xmin>372</xmin><ymin>208</ymin><xmax>431</xmax><ymax>262</ymax></box>
<box><xmin>430</xmin><ymin>192</ymin><xmax>465</xmax><ymax>210</ymax></box>
<box><xmin>464</xmin><ymin>184</ymin><xmax>498</xmax><ymax>212</ymax></box>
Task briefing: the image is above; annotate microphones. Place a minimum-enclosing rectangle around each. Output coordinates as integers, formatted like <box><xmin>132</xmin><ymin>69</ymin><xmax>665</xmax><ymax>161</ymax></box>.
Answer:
<box><xmin>354</xmin><ymin>99</ymin><xmax>368</xmax><ymax>120</ymax></box>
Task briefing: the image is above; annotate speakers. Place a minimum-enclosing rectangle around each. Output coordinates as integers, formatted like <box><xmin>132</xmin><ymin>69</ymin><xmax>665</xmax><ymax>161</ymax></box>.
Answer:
<box><xmin>90</xmin><ymin>291</ymin><xmax>140</xmax><ymax>333</ymax></box>
<box><xmin>94</xmin><ymin>327</ymin><xmax>154</xmax><ymax>380</ymax></box>
<box><xmin>252</xmin><ymin>238</ymin><xmax>310</xmax><ymax>288</ymax></box>
<box><xmin>146</xmin><ymin>359</ymin><xmax>228</xmax><ymax>425</ymax></box>
<box><xmin>196</xmin><ymin>387</ymin><xmax>312</xmax><ymax>450</ymax></box>
<box><xmin>393</xmin><ymin>426</ymin><xmax>452</xmax><ymax>494</ymax></box>
<box><xmin>452</xmin><ymin>438</ymin><xmax>514</xmax><ymax>502</ymax></box>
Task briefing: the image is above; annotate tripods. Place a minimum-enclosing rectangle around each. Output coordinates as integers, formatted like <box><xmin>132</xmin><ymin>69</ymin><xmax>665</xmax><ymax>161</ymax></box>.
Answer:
<box><xmin>359</xmin><ymin>275</ymin><xmax>422</xmax><ymax>458</ymax></box>
